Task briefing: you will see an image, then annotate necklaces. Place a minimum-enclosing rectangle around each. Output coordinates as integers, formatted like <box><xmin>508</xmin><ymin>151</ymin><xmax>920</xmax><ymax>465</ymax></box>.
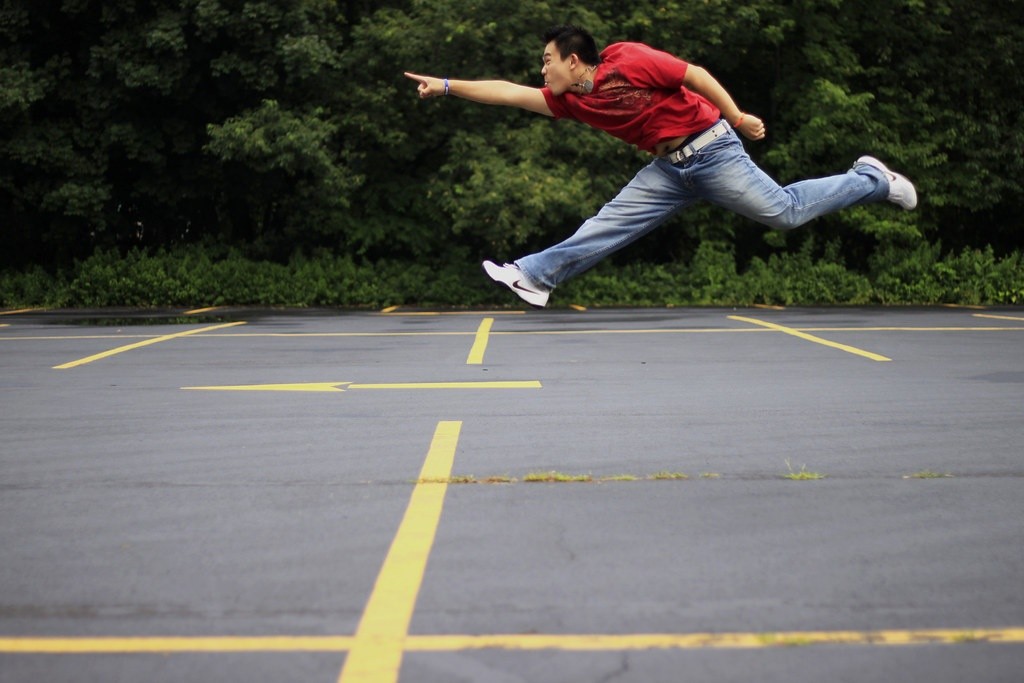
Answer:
<box><xmin>570</xmin><ymin>65</ymin><xmax>597</xmax><ymax>94</ymax></box>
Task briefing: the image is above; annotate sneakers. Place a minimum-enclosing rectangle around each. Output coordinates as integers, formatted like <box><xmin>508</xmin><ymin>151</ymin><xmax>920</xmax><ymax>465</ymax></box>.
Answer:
<box><xmin>857</xmin><ymin>155</ymin><xmax>918</xmax><ymax>210</ymax></box>
<box><xmin>482</xmin><ymin>260</ymin><xmax>549</xmax><ymax>310</ymax></box>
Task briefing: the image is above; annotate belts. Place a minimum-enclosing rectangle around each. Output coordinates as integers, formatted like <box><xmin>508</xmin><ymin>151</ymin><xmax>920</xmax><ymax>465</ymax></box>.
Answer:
<box><xmin>668</xmin><ymin>120</ymin><xmax>731</xmax><ymax>164</ymax></box>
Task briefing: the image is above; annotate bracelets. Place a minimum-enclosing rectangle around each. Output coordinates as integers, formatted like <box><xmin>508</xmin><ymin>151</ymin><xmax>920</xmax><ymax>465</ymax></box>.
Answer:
<box><xmin>445</xmin><ymin>79</ymin><xmax>449</xmax><ymax>96</ymax></box>
<box><xmin>733</xmin><ymin>112</ymin><xmax>744</xmax><ymax>128</ymax></box>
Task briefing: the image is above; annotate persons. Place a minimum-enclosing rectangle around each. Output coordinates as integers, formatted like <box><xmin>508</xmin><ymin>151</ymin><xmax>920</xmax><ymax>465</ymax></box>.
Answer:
<box><xmin>404</xmin><ymin>26</ymin><xmax>917</xmax><ymax>307</ymax></box>
